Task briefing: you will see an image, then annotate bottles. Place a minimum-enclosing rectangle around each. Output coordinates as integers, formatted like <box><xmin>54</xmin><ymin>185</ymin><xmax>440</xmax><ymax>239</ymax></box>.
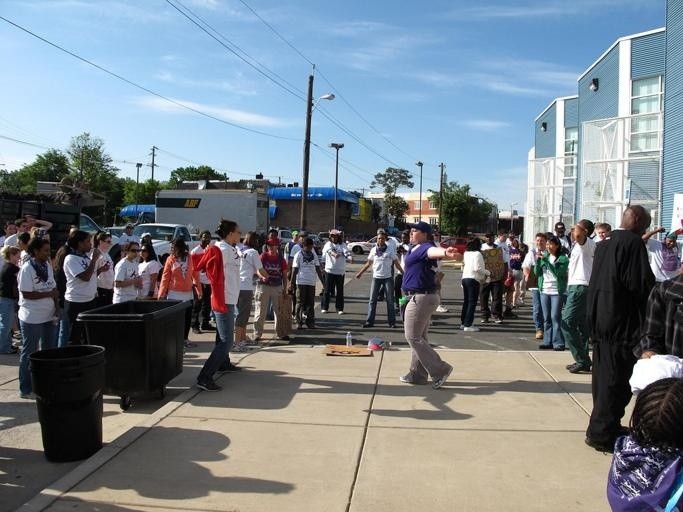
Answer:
<box><xmin>346</xmin><ymin>331</ymin><xmax>352</xmax><ymax>346</ymax></box>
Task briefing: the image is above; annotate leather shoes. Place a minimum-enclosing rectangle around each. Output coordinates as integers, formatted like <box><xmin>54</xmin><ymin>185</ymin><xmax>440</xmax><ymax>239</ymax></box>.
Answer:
<box><xmin>389</xmin><ymin>324</ymin><xmax>396</xmax><ymax>328</ymax></box>
<box><xmin>585</xmin><ymin>435</ymin><xmax>616</xmax><ymax>454</ymax></box>
<box><xmin>362</xmin><ymin>323</ymin><xmax>373</xmax><ymax>328</ymax></box>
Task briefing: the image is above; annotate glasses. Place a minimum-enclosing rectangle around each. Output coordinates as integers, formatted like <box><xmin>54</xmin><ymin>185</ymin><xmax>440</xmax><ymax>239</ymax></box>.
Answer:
<box><xmin>104</xmin><ymin>240</ymin><xmax>110</xmax><ymax>244</ymax></box>
<box><xmin>130</xmin><ymin>248</ymin><xmax>140</xmax><ymax>253</ymax></box>
<box><xmin>139</xmin><ymin>248</ymin><xmax>146</xmax><ymax>252</ymax></box>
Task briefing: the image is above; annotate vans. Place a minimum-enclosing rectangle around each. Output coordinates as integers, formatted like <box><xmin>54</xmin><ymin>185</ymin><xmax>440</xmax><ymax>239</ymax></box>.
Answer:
<box><xmin>155</xmin><ymin>233</ymin><xmax>198</xmax><ymax>241</ymax></box>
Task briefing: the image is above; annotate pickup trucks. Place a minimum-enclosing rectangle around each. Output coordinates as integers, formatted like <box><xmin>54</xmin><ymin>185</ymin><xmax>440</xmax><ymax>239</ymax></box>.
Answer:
<box><xmin>130</xmin><ymin>223</ymin><xmax>218</xmax><ymax>267</ymax></box>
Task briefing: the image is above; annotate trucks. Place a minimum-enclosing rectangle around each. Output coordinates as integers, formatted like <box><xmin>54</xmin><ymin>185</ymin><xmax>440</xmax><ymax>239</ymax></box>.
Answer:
<box><xmin>120</xmin><ymin>188</ymin><xmax>268</xmax><ymax>240</ymax></box>
<box><xmin>0</xmin><ymin>186</ymin><xmax>119</xmax><ymax>244</ymax></box>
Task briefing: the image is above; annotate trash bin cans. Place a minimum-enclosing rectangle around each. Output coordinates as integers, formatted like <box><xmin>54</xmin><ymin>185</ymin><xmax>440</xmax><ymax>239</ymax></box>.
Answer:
<box><xmin>75</xmin><ymin>299</ymin><xmax>193</xmax><ymax>411</ymax></box>
<box><xmin>27</xmin><ymin>345</ymin><xmax>106</xmax><ymax>463</ymax></box>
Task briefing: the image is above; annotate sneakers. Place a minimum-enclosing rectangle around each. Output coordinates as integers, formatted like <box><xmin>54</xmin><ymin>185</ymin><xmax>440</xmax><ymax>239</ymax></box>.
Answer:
<box><xmin>282</xmin><ymin>313</ymin><xmax>315</xmax><ymax>340</ymax></box>
<box><xmin>183</xmin><ymin>323</ymin><xmax>216</xmax><ymax>348</ymax></box>
<box><xmin>535</xmin><ymin>329</ymin><xmax>544</xmax><ymax>339</ymax></box>
<box><xmin>3</xmin><ymin>330</ymin><xmax>24</xmax><ymax>354</ymax></box>
<box><xmin>320</xmin><ymin>310</ymin><xmax>326</xmax><ymax>313</ymax></box>
<box><xmin>459</xmin><ymin>318</ymin><xmax>488</xmax><ymax>332</ymax></box>
<box><xmin>539</xmin><ymin>343</ymin><xmax>592</xmax><ymax>374</ymax></box>
<box><xmin>431</xmin><ymin>362</ymin><xmax>453</xmax><ymax>390</ymax></box>
<box><xmin>336</xmin><ymin>311</ymin><xmax>343</xmax><ymax>315</ymax></box>
<box><xmin>399</xmin><ymin>373</ymin><xmax>428</xmax><ymax>385</ymax></box>
<box><xmin>18</xmin><ymin>392</ymin><xmax>34</xmax><ymax>399</ymax></box>
<box><xmin>198</xmin><ymin>335</ymin><xmax>258</xmax><ymax>391</ymax></box>
<box><xmin>436</xmin><ymin>306</ymin><xmax>448</xmax><ymax>313</ymax></box>
<box><xmin>492</xmin><ymin>316</ymin><xmax>502</xmax><ymax>323</ymax></box>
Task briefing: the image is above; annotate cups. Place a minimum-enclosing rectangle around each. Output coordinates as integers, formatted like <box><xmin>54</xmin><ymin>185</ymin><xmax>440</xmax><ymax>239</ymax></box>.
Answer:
<box><xmin>384</xmin><ymin>338</ymin><xmax>391</xmax><ymax>349</ymax></box>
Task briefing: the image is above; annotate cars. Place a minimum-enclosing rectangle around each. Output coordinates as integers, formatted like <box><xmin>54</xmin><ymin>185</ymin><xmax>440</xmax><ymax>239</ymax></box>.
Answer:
<box><xmin>347</xmin><ymin>236</ymin><xmax>401</xmax><ymax>254</ymax></box>
<box><xmin>100</xmin><ymin>227</ymin><xmax>126</xmax><ymax>245</ymax></box>
<box><xmin>276</xmin><ymin>228</ymin><xmax>330</xmax><ymax>252</ymax></box>
<box><xmin>438</xmin><ymin>237</ymin><xmax>469</xmax><ymax>257</ymax></box>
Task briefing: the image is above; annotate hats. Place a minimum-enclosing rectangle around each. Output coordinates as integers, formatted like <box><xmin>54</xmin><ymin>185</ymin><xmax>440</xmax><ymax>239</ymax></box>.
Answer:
<box><xmin>555</xmin><ymin>221</ymin><xmax>566</xmax><ymax>233</ymax></box>
<box><xmin>264</xmin><ymin>229</ymin><xmax>342</xmax><ymax>245</ymax></box>
<box><xmin>406</xmin><ymin>222</ymin><xmax>432</xmax><ymax>234</ymax></box>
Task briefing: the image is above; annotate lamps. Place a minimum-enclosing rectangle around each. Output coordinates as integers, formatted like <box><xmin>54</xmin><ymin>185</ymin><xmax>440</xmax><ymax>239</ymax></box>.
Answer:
<box><xmin>540</xmin><ymin>122</ymin><xmax>546</xmax><ymax>132</ymax></box>
<box><xmin>588</xmin><ymin>77</ymin><xmax>598</xmax><ymax>91</ymax></box>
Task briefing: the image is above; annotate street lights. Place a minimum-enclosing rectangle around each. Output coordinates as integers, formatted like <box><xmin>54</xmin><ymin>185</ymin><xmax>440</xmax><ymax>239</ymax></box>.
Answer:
<box><xmin>132</xmin><ymin>163</ymin><xmax>143</xmax><ymax>222</ymax></box>
<box><xmin>299</xmin><ymin>75</ymin><xmax>337</xmax><ymax>229</ymax></box>
<box><xmin>416</xmin><ymin>161</ymin><xmax>423</xmax><ymax>225</ymax></box>
<box><xmin>510</xmin><ymin>201</ymin><xmax>518</xmax><ymax>233</ymax></box>
<box><xmin>328</xmin><ymin>142</ymin><xmax>344</xmax><ymax>229</ymax></box>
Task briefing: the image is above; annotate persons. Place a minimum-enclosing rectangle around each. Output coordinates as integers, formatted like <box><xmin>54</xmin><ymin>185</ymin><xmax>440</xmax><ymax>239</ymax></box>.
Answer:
<box><xmin>521</xmin><ymin>203</ymin><xmax>683</xmax><ymax>512</ymax></box>
<box><xmin>193</xmin><ymin>218</ymin><xmax>353</xmax><ymax>390</ymax></box>
<box><xmin>0</xmin><ymin>216</ymin><xmax>203</xmax><ymax>400</ymax></box>
<box><xmin>356</xmin><ymin>222</ymin><xmax>528</xmax><ymax>332</ymax></box>
<box><xmin>400</xmin><ymin>221</ymin><xmax>460</xmax><ymax>389</ymax></box>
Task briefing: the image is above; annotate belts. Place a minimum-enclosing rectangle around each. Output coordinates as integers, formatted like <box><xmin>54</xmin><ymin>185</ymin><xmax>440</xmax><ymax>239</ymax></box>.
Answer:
<box><xmin>407</xmin><ymin>290</ymin><xmax>436</xmax><ymax>294</ymax></box>
